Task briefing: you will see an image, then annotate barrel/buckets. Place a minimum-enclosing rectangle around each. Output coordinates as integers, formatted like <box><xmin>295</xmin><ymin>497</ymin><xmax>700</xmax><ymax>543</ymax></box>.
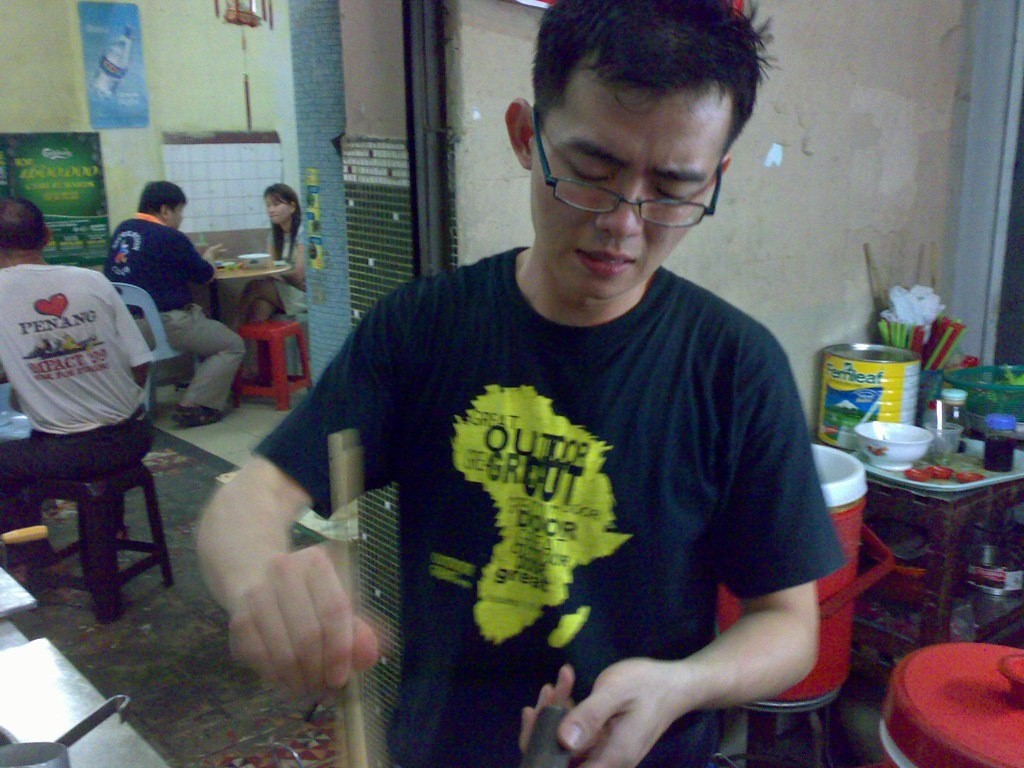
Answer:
<box><xmin>712</xmin><ymin>443</ymin><xmax>894</xmax><ymax>702</ymax></box>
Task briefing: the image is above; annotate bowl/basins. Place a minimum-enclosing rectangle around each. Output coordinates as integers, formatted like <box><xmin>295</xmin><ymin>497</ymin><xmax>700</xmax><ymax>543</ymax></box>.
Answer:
<box><xmin>854</xmin><ymin>422</ymin><xmax>933</xmax><ymax>471</ymax></box>
<box><xmin>238</xmin><ymin>253</ymin><xmax>271</xmax><ymax>268</ymax></box>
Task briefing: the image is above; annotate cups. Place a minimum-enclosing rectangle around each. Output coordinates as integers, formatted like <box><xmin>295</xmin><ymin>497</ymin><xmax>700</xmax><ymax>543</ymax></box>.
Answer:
<box><xmin>925</xmin><ymin>423</ymin><xmax>964</xmax><ymax>467</ymax></box>
<box><xmin>982</xmin><ymin>413</ymin><xmax>1017</xmax><ymax>473</ymax></box>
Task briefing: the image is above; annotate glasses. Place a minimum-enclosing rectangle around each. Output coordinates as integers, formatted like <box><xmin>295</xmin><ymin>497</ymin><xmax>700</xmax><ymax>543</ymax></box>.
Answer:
<box><xmin>532</xmin><ymin>104</ymin><xmax>721</xmax><ymax>227</ymax></box>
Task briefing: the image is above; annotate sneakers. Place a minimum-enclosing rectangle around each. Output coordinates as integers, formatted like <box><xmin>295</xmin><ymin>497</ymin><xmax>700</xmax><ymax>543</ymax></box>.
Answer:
<box><xmin>173</xmin><ymin>404</ymin><xmax>224</xmax><ymax>426</ymax></box>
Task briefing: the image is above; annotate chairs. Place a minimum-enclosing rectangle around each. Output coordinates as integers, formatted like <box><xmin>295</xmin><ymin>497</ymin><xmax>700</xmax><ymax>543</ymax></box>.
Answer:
<box><xmin>112</xmin><ymin>282</ymin><xmax>202</xmax><ymax>420</ymax></box>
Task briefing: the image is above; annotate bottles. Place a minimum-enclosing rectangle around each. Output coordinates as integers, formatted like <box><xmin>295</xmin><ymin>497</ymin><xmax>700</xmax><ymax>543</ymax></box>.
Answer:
<box><xmin>91</xmin><ymin>24</ymin><xmax>133</xmax><ymax>99</ymax></box>
<box><xmin>920</xmin><ymin>401</ymin><xmax>944</xmax><ymax>430</ymax></box>
<box><xmin>941</xmin><ymin>388</ymin><xmax>970</xmax><ymax>457</ymax></box>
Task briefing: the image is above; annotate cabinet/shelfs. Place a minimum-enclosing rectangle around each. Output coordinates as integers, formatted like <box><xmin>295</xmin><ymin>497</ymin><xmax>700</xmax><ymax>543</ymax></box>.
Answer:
<box><xmin>853</xmin><ymin>479</ymin><xmax>1024</xmax><ymax>657</ymax></box>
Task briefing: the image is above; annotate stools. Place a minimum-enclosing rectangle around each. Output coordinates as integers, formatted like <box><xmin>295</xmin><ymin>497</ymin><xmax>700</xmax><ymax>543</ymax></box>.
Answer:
<box><xmin>233</xmin><ymin>320</ymin><xmax>312</xmax><ymax>410</ymax></box>
<box><xmin>735</xmin><ymin>686</ymin><xmax>842</xmax><ymax>768</ymax></box>
<box><xmin>27</xmin><ymin>460</ymin><xmax>174</xmax><ymax>623</ymax></box>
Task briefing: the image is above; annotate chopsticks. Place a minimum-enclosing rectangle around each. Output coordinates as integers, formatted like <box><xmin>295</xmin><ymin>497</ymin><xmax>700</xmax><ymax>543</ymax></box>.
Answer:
<box><xmin>328</xmin><ymin>430</ymin><xmax>368</xmax><ymax>768</ymax></box>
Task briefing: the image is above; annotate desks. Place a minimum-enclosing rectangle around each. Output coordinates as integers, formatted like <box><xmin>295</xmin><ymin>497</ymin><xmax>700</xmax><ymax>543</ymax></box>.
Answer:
<box><xmin>210</xmin><ymin>257</ymin><xmax>293</xmax><ymax>318</ymax></box>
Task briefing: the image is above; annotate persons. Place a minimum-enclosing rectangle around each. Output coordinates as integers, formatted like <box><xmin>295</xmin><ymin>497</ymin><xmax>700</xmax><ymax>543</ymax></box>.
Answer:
<box><xmin>0</xmin><ymin>198</ymin><xmax>156</xmax><ymax>592</ymax></box>
<box><xmin>103</xmin><ymin>180</ymin><xmax>245</xmax><ymax>426</ymax></box>
<box><xmin>224</xmin><ymin>183</ymin><xmax>310</xmax><ymax>382</ymax></box>
<box><xmin>195</xmin><ymin>3</ymin><xmax>847</xmax><ymax>768</ymax></box>
